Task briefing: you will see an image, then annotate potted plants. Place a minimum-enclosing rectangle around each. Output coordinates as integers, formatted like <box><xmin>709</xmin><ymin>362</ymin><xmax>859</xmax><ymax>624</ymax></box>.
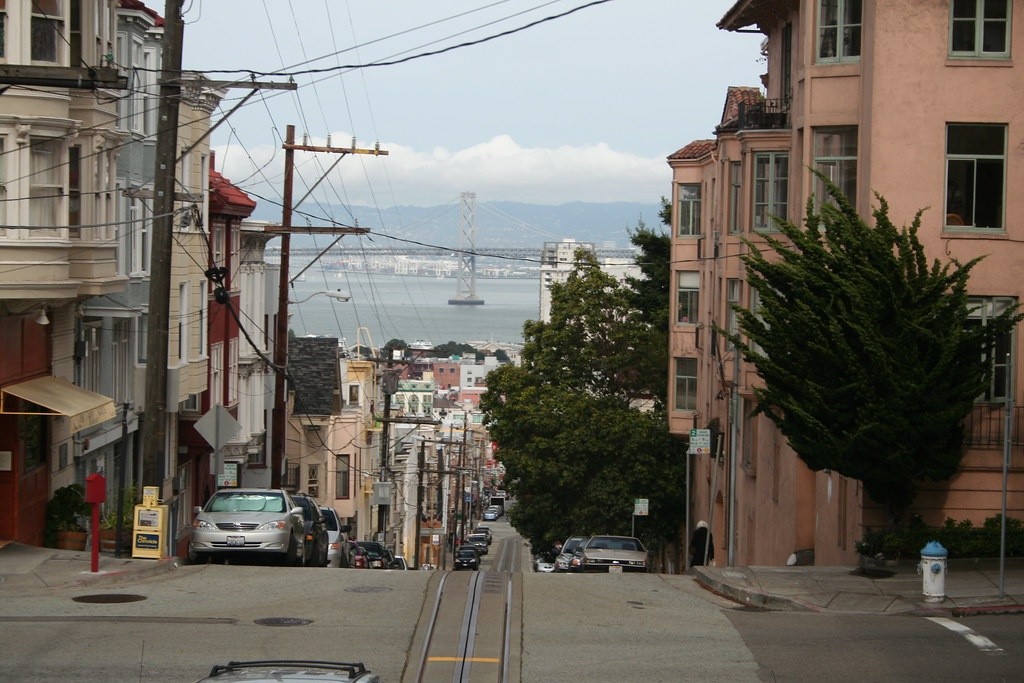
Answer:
<box><xmin>99</xmin><ymin>510</ymin><xmax>133</xmax><ymax>555</ymax></box>
<box><xmin>45</xmin><ymin>484</ymin><xmax>91</xmax><ymax>551</ymax></box>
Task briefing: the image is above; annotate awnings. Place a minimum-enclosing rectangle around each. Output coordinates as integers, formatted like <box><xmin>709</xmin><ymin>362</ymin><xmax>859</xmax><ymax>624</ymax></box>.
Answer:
<box><xmin>1</xmin><ymin>376</ymin><xmax>115</xmax><ymax>432</ymax></box>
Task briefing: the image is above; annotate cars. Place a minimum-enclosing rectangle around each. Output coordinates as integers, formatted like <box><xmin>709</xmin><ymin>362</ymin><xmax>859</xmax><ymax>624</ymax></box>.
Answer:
<box><xmin>532</xmin><ymin>534</ymin><xmax>650</xmax><ymax>574</ymax></box>
<box><xmin>482</xmin><ymin>505</ymin><xmax>503</xmax><ymax>521</ymax></box>
<box><xmin>453</xmin><ymin>527</ymin><xmax>492</xmax><ymax>570</ymax></box>
<box><xmin>188</xmin><ymin>488</ymin><xmax>409</xmax><ymax>570</ymax></box>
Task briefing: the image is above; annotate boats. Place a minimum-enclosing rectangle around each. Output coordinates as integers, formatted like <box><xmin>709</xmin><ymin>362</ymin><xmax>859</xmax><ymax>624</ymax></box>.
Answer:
<box><xmin>409</xmin><ymin>339</ymin><xmax>434</xmax><ymax>349</ymax></box>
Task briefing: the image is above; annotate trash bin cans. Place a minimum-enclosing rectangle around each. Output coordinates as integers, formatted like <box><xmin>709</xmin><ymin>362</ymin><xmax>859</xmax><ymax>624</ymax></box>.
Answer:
<box><xmin>133</xmin><ymin>487</ymin><xmax>170</xmax><ymax>559</ymax></box>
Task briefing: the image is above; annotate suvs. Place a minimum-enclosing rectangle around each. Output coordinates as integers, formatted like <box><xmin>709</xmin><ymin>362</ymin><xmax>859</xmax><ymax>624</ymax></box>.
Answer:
<box><xmin>193</xmin><ymin>659</ymin><xmax>381</xmax><ymax>683</ymax></box>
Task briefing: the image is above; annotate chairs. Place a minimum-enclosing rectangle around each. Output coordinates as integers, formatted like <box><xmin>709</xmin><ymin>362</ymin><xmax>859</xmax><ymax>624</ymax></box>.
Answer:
<box><xmin>594</xmin><ymin>542</ymin><xmax>608</xmax><ymax>548</ymax></box>
<box><xmin>622</xmin><ymin>544</ymin><xmax>635</xmax><ymax>551</ymax></box>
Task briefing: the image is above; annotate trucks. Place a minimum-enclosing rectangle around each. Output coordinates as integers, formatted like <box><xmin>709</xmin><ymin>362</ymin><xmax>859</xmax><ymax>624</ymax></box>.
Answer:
<box><xmin>491</xmin><ymin>489</ymin><xmax>509</xmax><ymax>505</ymax></box>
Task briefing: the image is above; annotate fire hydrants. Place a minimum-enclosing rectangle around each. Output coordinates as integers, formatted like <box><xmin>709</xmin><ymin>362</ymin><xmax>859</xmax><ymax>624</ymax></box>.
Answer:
<box><xmin>916</xmin><ymin>540</ymin><xmax>949</xmax><ymax>603</ymax></box>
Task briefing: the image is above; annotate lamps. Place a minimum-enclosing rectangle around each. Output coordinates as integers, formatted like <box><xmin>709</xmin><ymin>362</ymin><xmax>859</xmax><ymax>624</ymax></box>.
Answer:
<box><xmin>35</xmin><ymin>308</ymin><xmax>50</xmax><ymax>325</ymax></box>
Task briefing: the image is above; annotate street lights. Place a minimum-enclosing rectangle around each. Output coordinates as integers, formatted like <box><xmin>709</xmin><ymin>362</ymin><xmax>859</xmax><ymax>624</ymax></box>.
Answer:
<box><xmin>468</xmin><ymin>480</ymin><xmax>478</xmax><ymax>531</ymax></box>
<box><xmin>269</xmin><ymin>289</ymin><xmax>352</xmax><ymax>488</ymax></box>
